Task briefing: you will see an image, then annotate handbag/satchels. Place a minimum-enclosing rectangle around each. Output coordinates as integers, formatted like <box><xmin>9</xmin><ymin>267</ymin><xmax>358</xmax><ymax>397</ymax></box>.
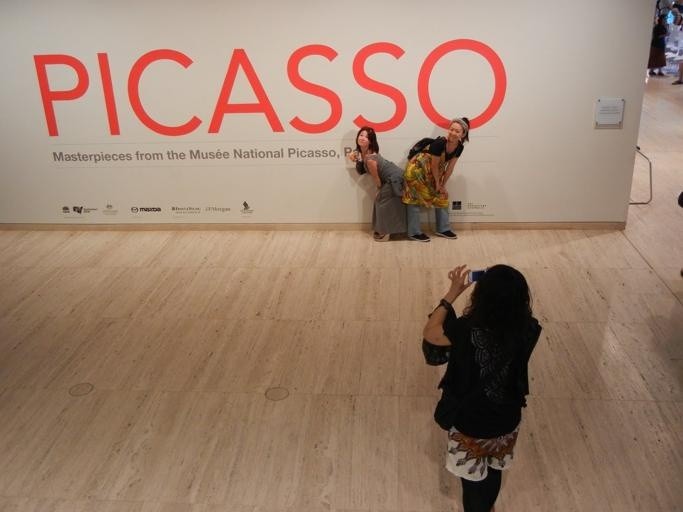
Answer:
<box><xmin>356</xmin><ymin>160</ymin><xmax>367</xmax><ymax>176</ymax></box>
<box><xmin>407</xmin><ymin>138</ymin><xmax>432</xmax><ymax>159</ymax></box>
<box><xmin>422</xmin><ymin>338</ymin><xmax>453</xmax><ymax>366</ymax></box>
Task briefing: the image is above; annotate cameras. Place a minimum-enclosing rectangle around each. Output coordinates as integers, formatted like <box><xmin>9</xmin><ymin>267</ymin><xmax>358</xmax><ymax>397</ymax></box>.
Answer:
<box><xmin>468</xmin><ymin>270</ymin><xmax>488</xmax><ymax>282</ymax></box>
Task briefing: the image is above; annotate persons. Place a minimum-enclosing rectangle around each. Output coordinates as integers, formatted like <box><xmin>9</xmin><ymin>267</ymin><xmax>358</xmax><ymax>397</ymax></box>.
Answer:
<box><xmin>648</xmin><ymin>14</ymin><xmax>671</xmax><ymax>75</ymax></box>
<box><xmin>401</xmin><ymin>118</ymin><xmax>470</xmax><ymax>242</ymax></box>
<box><xmin>672</xmin><ymin>60</ymin><xmax>683</xmax><ymax>85</ymax></box>
<box><xmin>349</xmin><ymin>126</ymin><xmax>407</xmax><ymax>239</ymax></box>
<box><xmin>422</xmin><ymin>265</ymin><xmax>542</xmax><ymax>512</ymax></box>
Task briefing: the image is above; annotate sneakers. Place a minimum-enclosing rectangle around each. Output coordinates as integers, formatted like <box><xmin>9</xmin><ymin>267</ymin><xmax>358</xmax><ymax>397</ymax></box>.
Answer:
<box><xmin>435</xmin><ymin>230</ymin><xmax>456</xmax><ymax>239</ymax></box>
<box><xmin>410</xmin><ymin>233</ymin><xmax>430</xmax><ymax>241</ymax></box>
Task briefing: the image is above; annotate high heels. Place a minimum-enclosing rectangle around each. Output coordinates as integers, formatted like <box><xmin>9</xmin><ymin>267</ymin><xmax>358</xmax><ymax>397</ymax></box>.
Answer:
<box><xmin>375</xmin><ymin>234</ymin><xmax>390</xmax><ymax>241</ymax></box>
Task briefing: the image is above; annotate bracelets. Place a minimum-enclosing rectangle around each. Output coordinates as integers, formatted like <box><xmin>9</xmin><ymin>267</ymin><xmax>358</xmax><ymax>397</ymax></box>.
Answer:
<box><xmin>376</xmin><ymin>185</ymin><xmax>382</xmax><ymax>189</ymax></box>
